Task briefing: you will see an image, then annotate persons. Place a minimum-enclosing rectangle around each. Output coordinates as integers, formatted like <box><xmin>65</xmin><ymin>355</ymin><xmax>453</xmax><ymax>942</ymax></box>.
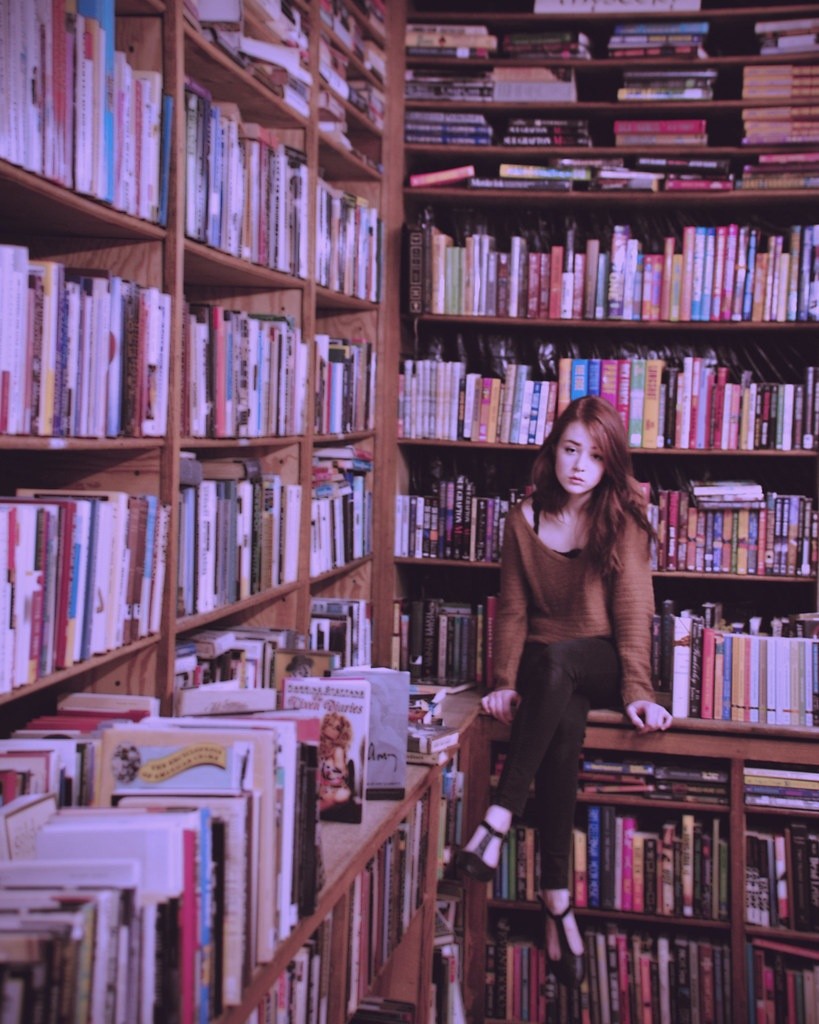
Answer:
<box><xmin>0</xmin><ymin>0</ymin><xmax>819</xmax><ymax>1024</ymax></box>
<box><xmin>316</xmin><ymin>699</ymin><xmax>362</xmax><ymax>806</ymax></box>
<box><xmin>459</xmin><ymin>396</ymin><xmax>672</xmax><ymax>993</ymax></box>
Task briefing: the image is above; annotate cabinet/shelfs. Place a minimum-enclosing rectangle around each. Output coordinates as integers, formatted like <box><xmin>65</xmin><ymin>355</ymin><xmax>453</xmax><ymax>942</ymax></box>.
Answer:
<box><xmin>0</xmin><ymin>0</ymin><xmax>819</xmax><ymax>1024</ymax></box>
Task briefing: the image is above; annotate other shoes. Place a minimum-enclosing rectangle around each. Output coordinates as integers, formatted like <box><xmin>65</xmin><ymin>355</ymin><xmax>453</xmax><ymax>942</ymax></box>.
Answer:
<box><xmin>451</xmin><ymin>817</ymin><xmax>507</xmax><ymax>883</ymax></box>
<box><xmin>534</xmin><ymin>892</ymin><xmax>588</xmax><ymax>988</ymax></box>
